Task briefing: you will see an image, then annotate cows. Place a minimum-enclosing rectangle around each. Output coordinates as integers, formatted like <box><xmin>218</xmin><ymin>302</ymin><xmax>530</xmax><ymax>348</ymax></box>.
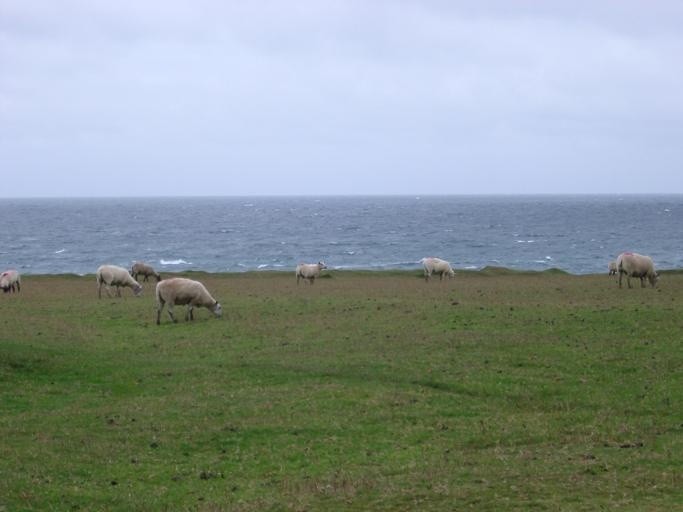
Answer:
<box><xmin>131</xmin><ymin>263</ymin><xmax>161</xmax><ymax>282</ymax></box>
<box><xmin>0</xmin><ymin>270</ymin><xmax>21</xmax><ymax>295</ymax></box>
<box><xmin>419</xmin><ymin>257</ymin><xmax>457</xmax><ymax>282</ymax></box>
<box><xmin>96</xmin><ymin>264</ymin><xmax>143</xmax><ymax>299</ymax></box>
<box><xmin>295</xmin><ymin>260</ymin><xmax>328</xmax><ymax>288</ymax></box>
<box><xmin>608</xmin><ymin>252</ymin><xmax>661</xmax><ymax>289</ymax></box>
<box><xmin>155</xmin><ymin>277</ymin><xmax>222</xmax><ymax>326</ymax></box>
